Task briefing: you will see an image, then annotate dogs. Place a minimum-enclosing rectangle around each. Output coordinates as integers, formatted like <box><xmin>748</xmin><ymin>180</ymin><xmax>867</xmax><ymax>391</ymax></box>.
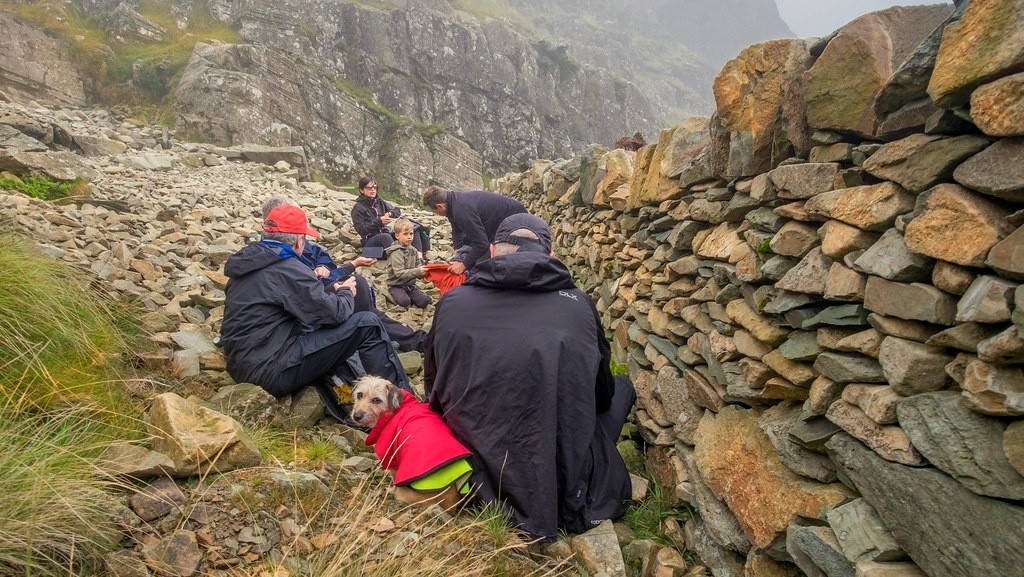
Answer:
<box><xmin>351</xmin><ymin>375</ymin><xmax>472</xmax><ymax>514</ymax></box>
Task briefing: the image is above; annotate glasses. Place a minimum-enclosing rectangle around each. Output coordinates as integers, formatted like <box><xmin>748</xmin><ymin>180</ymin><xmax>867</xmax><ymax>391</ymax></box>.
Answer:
<box><xmin>364</xmin><ymin>184</ymin><xmax>377</xmax><ymax>190</ymax></box>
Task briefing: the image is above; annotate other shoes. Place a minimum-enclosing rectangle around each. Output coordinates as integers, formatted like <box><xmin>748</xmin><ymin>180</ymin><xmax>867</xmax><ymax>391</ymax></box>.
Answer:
<box><xmin>418</xmin><ymin>252</ymin><xmax>430</xmax><ymax>265</ymax></box>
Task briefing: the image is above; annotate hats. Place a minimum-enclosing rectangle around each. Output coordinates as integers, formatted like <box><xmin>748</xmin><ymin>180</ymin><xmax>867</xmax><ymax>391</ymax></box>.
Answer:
<box><xmin>492</xmin><ymin>213</ymin><xmax>551</xmax><ymax>253</ymax></box>
<box><xmin>261</xmin><ymin>204</ymin><xmax>320</xmax><ymax>237</ymax></box>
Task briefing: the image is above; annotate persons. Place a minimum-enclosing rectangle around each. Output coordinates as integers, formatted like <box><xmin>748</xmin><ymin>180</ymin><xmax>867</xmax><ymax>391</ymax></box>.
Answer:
<box><xmin>384</xmin><ymin>218</ymin><xmax>433</xmax><ymax>307</ymax></box>
<box><xmin>422</xmin><ymin>186</ymin><xmax>528</xmax><ymax>275</ymax></box>
<box><xmin>220</xmin><ymin>194</ymin><xmax>429</xmax><ymax>396</ymax></box>
<box><xmin>351</xmin><ymin>176</ymin><xmax>423</xmax><ymax>252</ymax></box>
<box><xmin>424</xmin><ymin>213</ymin><xmax>636</xmax><ymax>544</ymax></box>
